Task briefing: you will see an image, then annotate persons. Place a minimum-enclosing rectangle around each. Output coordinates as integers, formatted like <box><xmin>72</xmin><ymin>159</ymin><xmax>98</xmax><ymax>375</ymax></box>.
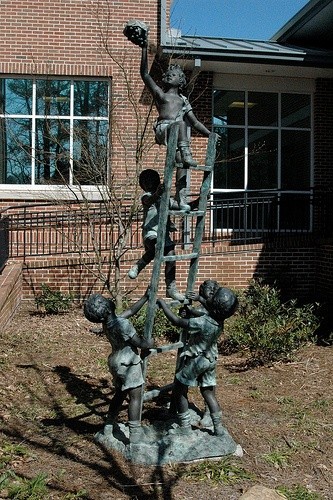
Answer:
<box><xmin>85</xmin><ymin>284</ymin><xmax>157</xmax><ymax>444</ymax></box>
<box><xmin>128</xmin><ymin>168</ymin><xmax>186</xmax><ymax>302</ymax></box>
<box><xmin>181</xmin><ymin>278</ymin><xmax>224</xmax><ymax>427</ymax></box>
<box><xmin>139</xmin><ymin>27</ymin><xmax>222</xmax><ymax>212</ymax></box>
<box><xmin>156</xmin><ymin>285</ymin><xmax>240</xmax><ymax>436</ymax></box>
